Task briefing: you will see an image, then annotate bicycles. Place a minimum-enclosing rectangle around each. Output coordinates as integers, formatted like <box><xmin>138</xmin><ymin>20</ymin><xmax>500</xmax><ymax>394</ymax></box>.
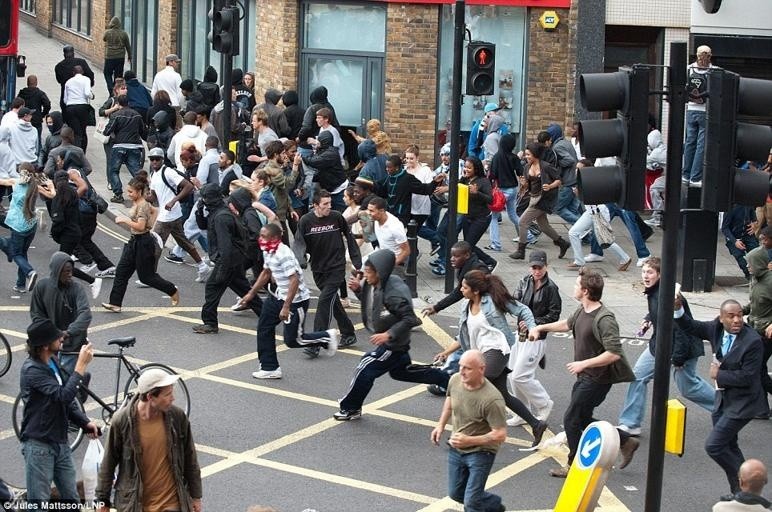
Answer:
<box><xmin>12</xmin><ymin>335</ymin><xmax>192</xmax><ymax>452</ymax></box>
<box><xmin>1</xmin><ymin>332</ymin><xmax>12</xmax><ymax>379</ymax></box>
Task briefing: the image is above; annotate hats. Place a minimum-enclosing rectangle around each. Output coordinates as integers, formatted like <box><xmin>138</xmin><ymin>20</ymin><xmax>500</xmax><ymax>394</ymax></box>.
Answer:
<box><xmin>18</xmin><ymin>107</ymin><xmax>36</xmax><ymax>117</ymax></box>
<box><xmin>26</xmin><ymin>318</ymin><xmax>64</xmax><ymax>347</ymax></box>
<box><xmin>696</xmin><ymin>45</ymin><xmax>712</xmax><ymax>56</ymax></box>
<box><xmin>484</xmin><ymin>103</ymin><xmax>501</xmax><ymax>113</ymax></box>
<box><xmin>138</xmin><ymin>369</ymin><xmax>182</xmax><ymax>394</ymax></box>
<box><xmin>529</xmin><ymin>250</ymin><xmax>547</xmax><ymax>267</ymax></box>
<box><xmin>165</xmin><ymin>54</ymin><xmax>181</xmax><ymax>62</ymax></box>
<box><xmin>180</xmin><ymin>80</ymin><xmax>193</xmax><ymax>92</ymax></box>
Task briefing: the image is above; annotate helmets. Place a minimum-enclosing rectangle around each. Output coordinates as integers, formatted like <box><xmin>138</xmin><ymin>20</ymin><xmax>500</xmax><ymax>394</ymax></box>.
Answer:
<box><xmin>147</xmin><ymin>147</ymin><xmax>165</xmax><ymax>158</ymax></box>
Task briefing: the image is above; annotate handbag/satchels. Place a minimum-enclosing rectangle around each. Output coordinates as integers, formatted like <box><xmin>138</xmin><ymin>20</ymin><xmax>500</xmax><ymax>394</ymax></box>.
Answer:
<box><xmin>86</xmin><ymin>192</ymin><xmax>108</xmax><ymax>213</ymax></box>
<box><xmin>88</xmin><ymin>104</ymin><xmax>110</xmax><ymax>144</ymax></box>
<box><xmin>593</xmin><ymin>204</ymin><xmax>615</xmax><ymax>248</ymax></box>
<box><xmin>487</xmin><ymin>186</ymin><xmax>506</xmax><ymax>211</ymax></box>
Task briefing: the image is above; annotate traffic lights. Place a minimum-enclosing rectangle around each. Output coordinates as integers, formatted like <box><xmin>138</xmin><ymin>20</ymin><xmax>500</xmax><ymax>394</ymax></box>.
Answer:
<box><xmin>572</xmin><ymin>64</ymin><xmax>651</xmax><ymax>214</ymax></box>
<box><xmin>214</xmin><ymin>9</ymin><xmax>234</xmax><ymax>52</ymax></box>
<box><xmin>468</xmin><ymin>39</ymin><xmax>496</xmax><ymax>95</ymax></box>
<box><xmin>207</xmin><ymin>7</ymin><xmax>216</xmax><ymax>43</ymax></box>
<box><xmin>698</xmin><ymin>61</ymin><xmax>771</xmax><ymax>213</ymax></box>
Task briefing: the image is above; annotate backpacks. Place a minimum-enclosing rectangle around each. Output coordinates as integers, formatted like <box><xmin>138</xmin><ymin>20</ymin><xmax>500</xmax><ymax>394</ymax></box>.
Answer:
<box><xmin>215</xmin><ymin>209</ymin><xmax>260</xmax><ymax>261</ymax></box>
<box><xmin>688</xmin><ymin>69</ymin><xmax>708</xmax><ymax>103</ymax></box>
<box><xmin>162</xmin><ymin>163</ymin><xmax>190</xmax><ymax>203</ymax></box>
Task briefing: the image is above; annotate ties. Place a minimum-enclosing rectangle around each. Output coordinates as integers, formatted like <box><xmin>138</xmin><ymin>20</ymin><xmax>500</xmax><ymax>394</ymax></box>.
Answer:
<box><xmin>721</xmin><ymin>334</ymin><xmax>733</xmax><ymax>358</ymax></box>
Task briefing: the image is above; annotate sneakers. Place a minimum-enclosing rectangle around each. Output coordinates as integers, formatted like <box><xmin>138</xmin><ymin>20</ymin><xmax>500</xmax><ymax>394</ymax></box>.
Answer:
<box><xmin>192</xmin><ymin>324</ymin><xmax>218</xmax><ymax>334</ymax></box>
<box><xmin>12</xmin><ymin>270</ymin><xmax>38</xmax><ymax>293</ymax></box>
<box><xmin>333</xmin><ymin>409</ymin><xmax>361</xmax><ymax>420</ymax></box>
<box><xmin>108</xmin><ymin>183</ymin><xmax>124</xmax><ymax>202</ymax></box>
<box><xmin>79</xmin><ymin>262</ymin><xmax>121</xmax><ymax>312</ymax></box>
<box><xmin>171</xmin><ymin>286</ymin><xmax>179</xmax><ymax>307</ymax></box>
<box><xmin>488</xmin><ymin>258</ymin><xmax>499</xmax><ymax>273</ymax></box>
<box><xmin>613</xmin><ymin>424</ymin><xmax>642</xmax><ymax>469</ymax></box>
<box><xmin>617</xmin><ymin>254</ymin><xmax>652</xmax><ymax>270</ymax></box>
<box><xmin>506</xmin><ymin>400</ymin><xmax>554</xmax><ymax>447</ymax></box>
<box><xmin>427</xmin><ymin>386</ymin><xmax>446</xmax><ymax>395</ymax></box>
<box><xmin>135</xmin><ymin>280</ymin><xmax>150</xmax><ymax>288</ymax></box>
<box><xmin>163</xmin><ymin>254</ymin><xmax>212</xmax><ymax>283</ymax></box>
<box><xmin>484</xmin><ymin>216</ymin><xmax>542</xmax><ymax>259</ymax></box>
<box><xmin>553</xmin><ymin>236</ymin><xmax>604</xmax><ymax>267</ymax></box>
<box><xmin>429</xmin><ymin>231</ymin><xmax>446</xmax><ymax>274</ymax></box>
<box><xmin>303</xmin><ymin>328</ymin><xmax>357</xmax><ymax>357</ymax></box>
<box><xmin>252</xmin><ymin>367</ymin><xmax>283</xmax><ymax>379</ymax></box>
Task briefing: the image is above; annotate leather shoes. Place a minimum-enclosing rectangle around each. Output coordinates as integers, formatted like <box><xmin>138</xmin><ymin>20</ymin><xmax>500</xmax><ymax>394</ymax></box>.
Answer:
<box><xmin>548</xmin><ymin>464</ymin><xmax>571</xmax><ymax>478</ymax></box>
<box><xmin>720</xmin><ymin>494</ymin><xmax>735</xmax><ymax>501</ymax></box>
